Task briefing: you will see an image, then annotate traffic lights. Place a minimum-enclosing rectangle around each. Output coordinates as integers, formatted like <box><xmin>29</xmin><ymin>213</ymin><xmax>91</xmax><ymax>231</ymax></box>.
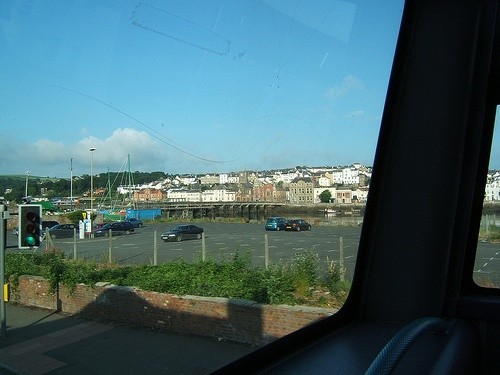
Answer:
<box><xmin>17</xmin><ymin>205</ymin><xmax>43</xmax><ymax>249</ymax></box>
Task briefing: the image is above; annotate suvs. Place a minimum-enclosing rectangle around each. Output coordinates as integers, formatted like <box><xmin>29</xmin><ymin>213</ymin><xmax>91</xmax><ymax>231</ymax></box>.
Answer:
<box><xmin>264</xmin><ymin>217</ymin><xmax>287</xmax><ymax>231</ymax></box>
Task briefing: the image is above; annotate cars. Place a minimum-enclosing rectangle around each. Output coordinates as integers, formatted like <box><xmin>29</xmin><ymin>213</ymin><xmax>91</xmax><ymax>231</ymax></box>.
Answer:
<box><xmin>285</xmin><ymin>219</ymin><xmax>312</xmax><ymax>232</ymax></box>
<box><xmin>85</xmin><ymin>218</ymin><xmax>144</xmax><ymax>237</ymax></box>
<box><xmin>41</xmin><ymin>223</ymin><xmax>79</xmax><ymax>239</ymax></box>
<box><xmin>160</xmin><ymin>224</ymin><xmax>204</xmax><ymax>242</ymax></box>
<box><xmin>42</xmin><ymin>221</ymin><xmax>59</xmax><ymax>231</ymax></box>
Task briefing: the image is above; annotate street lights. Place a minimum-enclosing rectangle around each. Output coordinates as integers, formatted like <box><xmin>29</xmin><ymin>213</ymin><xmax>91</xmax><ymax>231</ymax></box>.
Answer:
<box><xmin>89</xmin><ymin>148</ymin><xmax>96</xmax><ymax>234</ymax></box>
<box><xmin>70</xmin><ymin>156</ymin><xmax>76</xmax><ymax>211</ymax></box>
